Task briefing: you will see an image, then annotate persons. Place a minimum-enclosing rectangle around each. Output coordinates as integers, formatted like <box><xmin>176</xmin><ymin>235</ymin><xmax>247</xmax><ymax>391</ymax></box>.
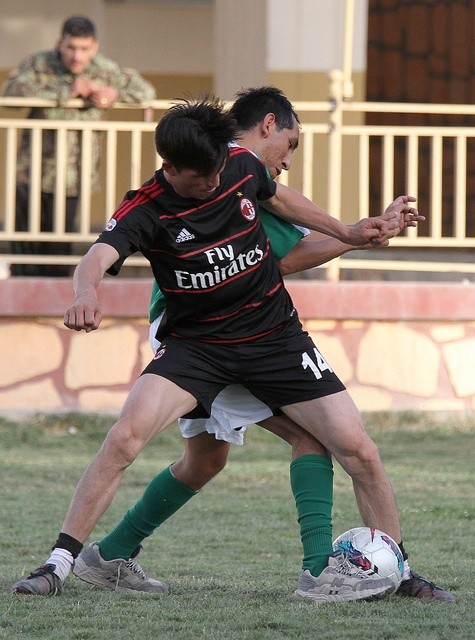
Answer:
<box><xmin>5</xmin><ymin>14</ymin><xmax>156</xmax><ymax>277</ymax></box>
<box><xmin>12</xmin><ymin>93</ymin><xmax>457</xmax><ymax>607</ymax></box>
<box><xmin>73</xmin><ymin>83</ymin><xmax>427</xmax><ymax>597</ymax></box>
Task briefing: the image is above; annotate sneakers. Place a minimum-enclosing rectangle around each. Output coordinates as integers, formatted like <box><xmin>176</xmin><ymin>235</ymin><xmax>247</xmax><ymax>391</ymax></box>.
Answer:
<box><xmin>398</xmin><ymin>570</ymin><xmax>456</xmax><ymax>601</ymax></box>
<box><xmin>72</xmin><ymin>541</ymin><xmax>168</xmax><ymax>596</ymax></box>
<box><xmin>294</xmin><ymin>551</ymin><xmax>396</xmax><ymax>602</ymax></box>
<box><xmin>12</xmin><ymin>563</ymin><xmax>63</xmax><ymax>596</ymax></box>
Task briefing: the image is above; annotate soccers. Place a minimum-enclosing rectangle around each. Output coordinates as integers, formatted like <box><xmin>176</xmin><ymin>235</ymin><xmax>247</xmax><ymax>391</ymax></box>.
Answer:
<box><xmin>332</xmin><ymin>527</ymin><xmax>404</xmax><ymax>599</ymax></box>
